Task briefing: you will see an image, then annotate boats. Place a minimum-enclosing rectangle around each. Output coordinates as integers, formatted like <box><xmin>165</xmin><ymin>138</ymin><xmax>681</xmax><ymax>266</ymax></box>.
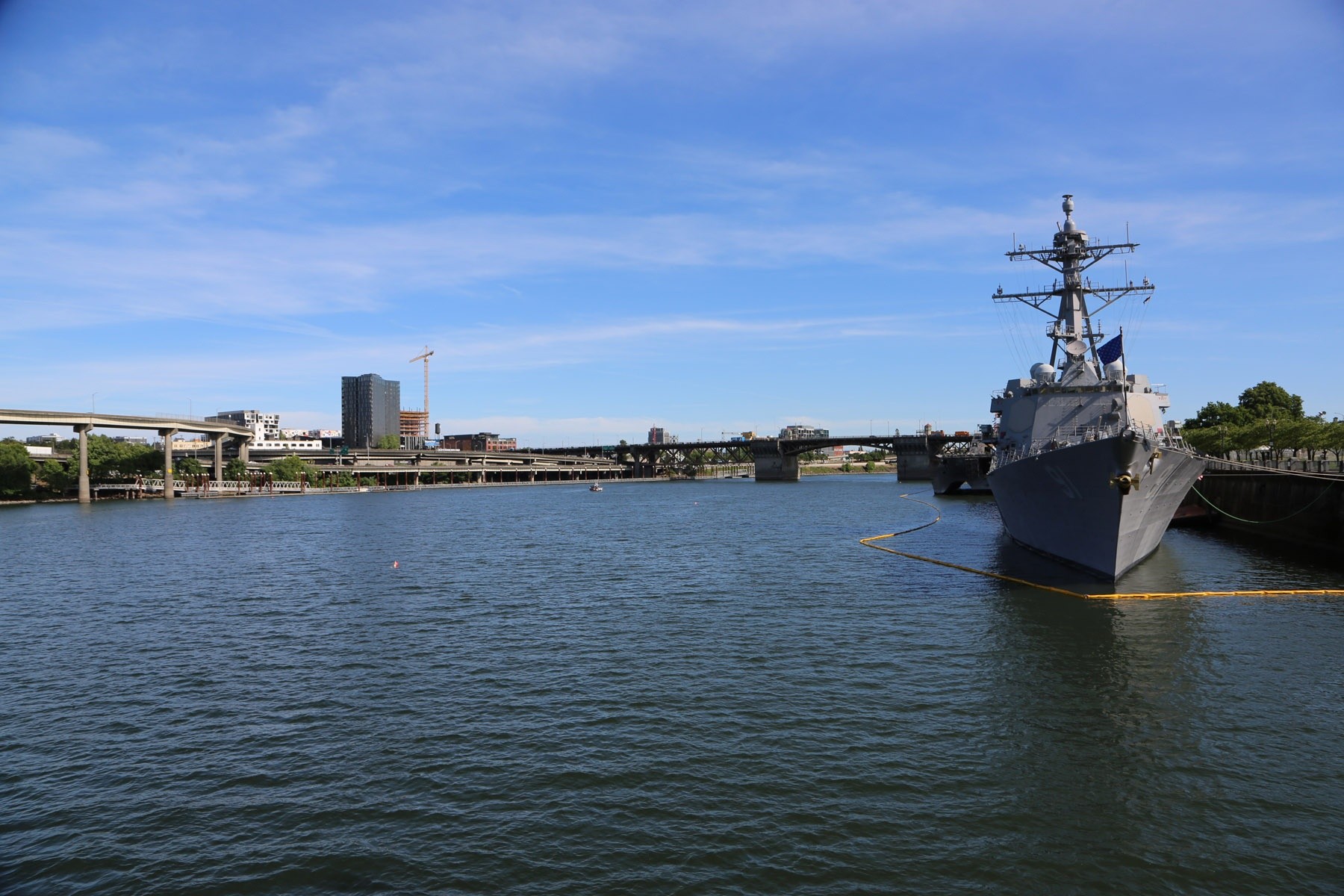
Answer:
<box><xmin>987</xmin><ymin>194</ymin><xmax>1211</xmax><ymax>585</ymax></box>
<box><xmin>589</xmin><ymin>485</ymin><xmax>604</xmax><ymax>491</ymax></box>
<box><xmin>932</xmin><ymin>431</ymin><xmax>993</xmax><ymax>493</ymax></box>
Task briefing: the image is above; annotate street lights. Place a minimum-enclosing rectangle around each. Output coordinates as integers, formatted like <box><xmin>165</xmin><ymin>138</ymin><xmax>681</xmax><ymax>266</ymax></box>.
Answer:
<box><xmin>919</xmin><ymin>419</ymin><xmax>921</xmax><ymax>431</ymax></box>
<box><xmin>795</xmin><ymin>421</ymin><xmax>797</xmax><ymax>441</ymax></box>
<box><xmin>455</xmin><ymin>440</ymin><xmax>460</xmax><ymax>449</ymax></box>
<box><xmin>446</xmin><ymin>443</ymin><xmax>452</xmax><ymax>450</ymax></box>
<box><xmin>870</xmin><ymin>419</ymin><xmax>873</xmax><ymax>436</ymax></box>
<box><xmin>92</xmin><ymin>391</ymin><xmax>100</xmax><ymax>414</ymax></box>
<box><xmin>1265</xmin><ymin>419</ymin><xmax>1277</xmax><ymax>461</ymax></box>
<box><xmin>364</xmin><ymin>433</ymin><xmax>370</xmax><ymax>465</ymax></box>
<box><xmin>1218</xmin><ymin>426</ymin><xmax>1228</xmax><ymax>459</ymax></box>
<box><xmin>888</xmin><ymin>419</ymin><xmax>890</xmax><ymax>437</ymax></box>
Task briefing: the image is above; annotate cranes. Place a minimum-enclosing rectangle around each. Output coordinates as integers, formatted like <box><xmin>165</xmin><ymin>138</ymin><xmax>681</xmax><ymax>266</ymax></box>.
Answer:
<box><xmin>407</xmin><ymin>344</ymin><xmax>435</xmax><ymax>439</ymax></box>
